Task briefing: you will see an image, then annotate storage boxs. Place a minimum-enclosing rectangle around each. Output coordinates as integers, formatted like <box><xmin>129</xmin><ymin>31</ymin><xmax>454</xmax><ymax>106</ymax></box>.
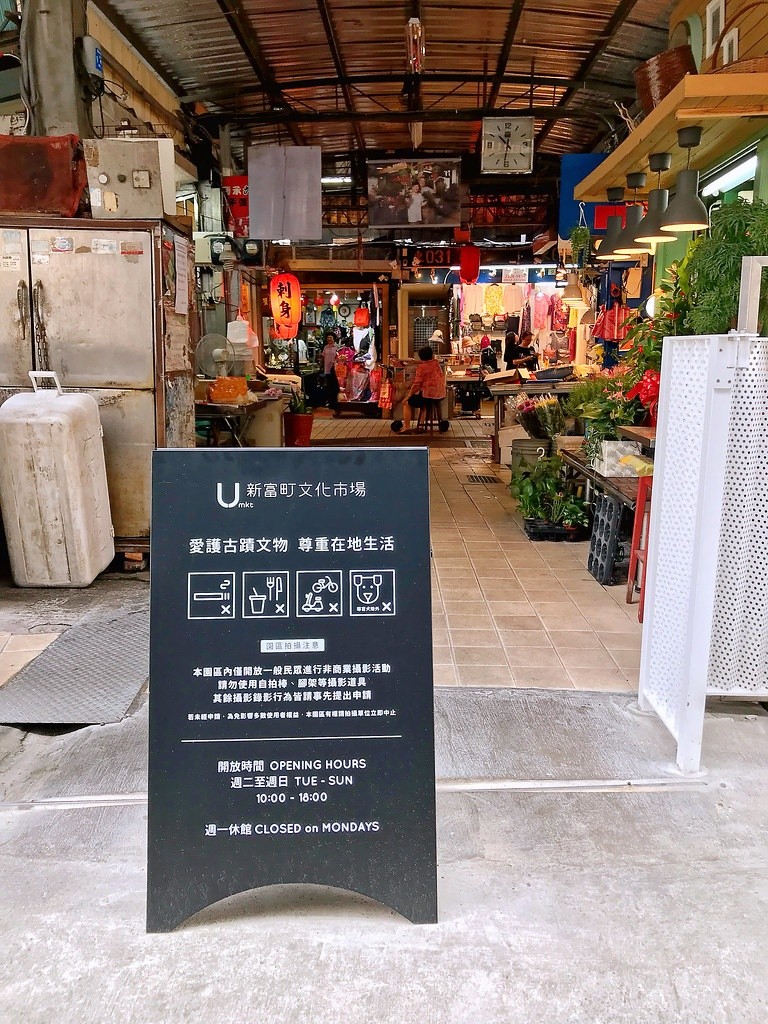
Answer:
<box><xmin>497</xmin><ymin>424</ymin><xmax>530</xmax><ymax>465</ymax></box>
<box><xmin>0</xmin><ymin>369</ymin><xmax>115</xmax><ymax>588</ymax></box>
<box><xmin>483</xmin><ymin>369</ymin><xmax>529</xmax><ymax>386</ymax></box>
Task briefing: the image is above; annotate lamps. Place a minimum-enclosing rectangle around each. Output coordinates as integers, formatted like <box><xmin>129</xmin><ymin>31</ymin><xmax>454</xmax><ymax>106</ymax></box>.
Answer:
<box><xmin>283</xmin><ymin>386</ymin><xmax>314</xmax><ymax>448</ymax></box>
<box><xmin>195</xmin><ymin>333</ymin><xmax>236</xmax><ymax>376</ymax></box>
<box><xmin>613</xmin><ymin>171</ymin><xmax>651</xmax><ymax>255</ymax></box>
<box><xmin>660</xmin><ymin>126</ymin><xmax>709</xmax><ymax>232</ymax></box>
<box><xmin>634</xmin><ymin>153</ymin><xmax>677</xmax><ymax>243</ymax></box>
<box><xmin>414</xmin><ymin>268</ymin><xmax>420</xmax><ymax>279</ymax></box>
<box><xmin>430</xmin><ymin>269</ymin><xmax>438</xmax><ymax>285</ymax></box>
<box><xmin>406</xmin><ymin>17</ymin><xmax>426</xmax><ymax>74</ymax></box>
<box><xmin>596</xmin><ymin>189</ymin><xmax>632</xmax><ymax>260</ymax></box>
<box><xmin>560</xmin><ymin>265</ymin><xmax>591</xmax><ymax>310</ymax></box>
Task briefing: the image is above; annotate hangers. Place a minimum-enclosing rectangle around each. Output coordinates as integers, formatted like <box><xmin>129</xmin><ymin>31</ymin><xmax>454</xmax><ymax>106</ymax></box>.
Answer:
<box><xmin>537</xmin><ymin>287</ymin><xmax>544</xmax><ymax>295</ymax></box>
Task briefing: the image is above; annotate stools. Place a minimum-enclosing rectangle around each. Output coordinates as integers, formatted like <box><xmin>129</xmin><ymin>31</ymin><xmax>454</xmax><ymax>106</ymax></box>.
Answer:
<box><xmin>416</xmin><ymin>405</ymin><xmax>443</xmax><ymax>437</ymax></box>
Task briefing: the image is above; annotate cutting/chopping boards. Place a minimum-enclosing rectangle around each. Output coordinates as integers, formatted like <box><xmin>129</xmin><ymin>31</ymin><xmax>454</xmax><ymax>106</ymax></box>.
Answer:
<box><xmin>254</xmin><ymin>391</ymin><xmax>282</xmax><ymax>399</ymax></box>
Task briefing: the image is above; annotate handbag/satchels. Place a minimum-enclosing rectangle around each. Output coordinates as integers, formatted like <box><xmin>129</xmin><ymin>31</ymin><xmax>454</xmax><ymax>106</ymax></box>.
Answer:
<box><xmin>378</xmin><ymin>378</ymin><xmax>397</xmax><ymax>409</ymax></box>
<box><xmin>494</xmin><ymin>312</ymin><xmax>509</xmax><ymax>322</ymax></box>
<box><xmin>469</xmin><ymin>313</ymin><xmax>481</xmax><ymax>322</ymax></box>
<box><xmin>543</xmin><ymin>343</ymin><xmax>557</xmax><ymax>364</ymax></box>
<box><xmin>354</xmin><ymin>300</ymin><xmax>369</xmax><ymax>326</ymax></box>
<box><xmin>454</xmin><ymin>381</ymin><xmax>493</xmax><ymax>411</ymax></box>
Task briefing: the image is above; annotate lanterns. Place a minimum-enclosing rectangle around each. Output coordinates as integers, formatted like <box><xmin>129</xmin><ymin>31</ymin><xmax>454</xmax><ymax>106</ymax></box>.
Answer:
<box><xmin>330</xmin><ymin>295</ymin><xmax>340</xmax><ymax>311</ymax></box>
<box><xmin>354</xmin><ymin>308</ymin><xmax>369</xmax><ymax>327</ymax></box>
<box><xmin>460</xmin><ymin>246</ymin><xmax>480</xmax><ymax>282</ymax></box>
<box><xmin>313</xmin><ymin>296</ymin><xmax>323</xmax><ymax>311</ymax></box>
<box><xmin>274</xmin><ymin>319</ymin><xmax>298</xmax><ymax>341</ymax></box>
<box><xmin>454</xmin><ymin>223</ymin><xmax>471</xmax><ymax>243</ymax></box>
<box><xmin>459</xmin><ymin>271</ymin><xmax>479</xmax><ymax>285</ymax></box>
<box><xmin>270</xmin><ymin>274</ymin><xmax>301</xmax><ymax>328</ymax></box>
<box><xmin>302</xmin><ymin>295</ymin><xmax>309</xmax><ymax>311</ymax></box>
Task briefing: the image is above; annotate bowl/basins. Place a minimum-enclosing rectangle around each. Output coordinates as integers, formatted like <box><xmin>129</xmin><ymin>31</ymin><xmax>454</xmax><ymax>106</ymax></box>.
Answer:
<box><xmin>535</xmin><ymin>366</ymin><xmax>574</xmax><ymax>379</ymax></box>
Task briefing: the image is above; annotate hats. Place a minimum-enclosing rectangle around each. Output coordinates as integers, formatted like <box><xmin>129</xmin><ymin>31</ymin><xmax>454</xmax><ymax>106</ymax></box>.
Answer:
<box><xmin>461</xmin><ymin>336</ymin><xmax>476</xmax><ymax>348</ymax></box>
<box><xmin>428</xmin><ymin>329</ymin><xmax>445</xmax><ymax>345</ymax></box>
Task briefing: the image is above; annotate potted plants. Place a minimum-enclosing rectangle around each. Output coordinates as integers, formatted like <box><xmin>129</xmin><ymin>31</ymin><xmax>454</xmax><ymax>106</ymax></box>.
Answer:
<box><xmin>505</xmin><ymin>456</ymin><xmax>591</xmax><ymax>541</ymax></box>
<box><xmin>570</xmin><ymin>226</ymin><xmax>592</xmax><ymax>269</ymax></box>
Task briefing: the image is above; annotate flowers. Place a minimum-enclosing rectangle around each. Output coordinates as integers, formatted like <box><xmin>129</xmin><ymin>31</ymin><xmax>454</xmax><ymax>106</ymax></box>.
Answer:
<box><xmin>507</xmin><ymin>388</ymin><xmax>566</xmax><ymax>438</ymax></box>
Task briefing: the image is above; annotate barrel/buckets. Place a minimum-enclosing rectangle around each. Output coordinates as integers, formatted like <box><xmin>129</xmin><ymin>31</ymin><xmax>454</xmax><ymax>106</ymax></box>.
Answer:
<box><xmin>511</xmin><ymin>439</ymin><xmax>550</xmax><ymax>483</ymax></box>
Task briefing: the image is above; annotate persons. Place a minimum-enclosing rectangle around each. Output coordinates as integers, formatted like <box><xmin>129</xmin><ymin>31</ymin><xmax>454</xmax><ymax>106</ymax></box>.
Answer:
<box><xmin>321</xmin><ymin>332</ymin><xmax>339</xmax><ymax>409</ymax></box>
<box><xmin>397</xmin><ymin>346</ymin><xmax>446</xmax><ymax>434</ymax></box>
<box><xmin>507</xmin><ymin>331</ymin><xmax>538</xmax><ymax>371</ymax></box>
<box><xmin>406</xmin><ymin>181</ymin><xmax>424</xmax><ymax>225</ymax></box>
<box><xmin>504</xmin><ymin>332</ymin><xmax>519</xmax><ymax>361</ymax></box>
<box><xmin>417</xmin><ymin>177</ymin><xmax>435</xmax><ymax>225</ymax></box>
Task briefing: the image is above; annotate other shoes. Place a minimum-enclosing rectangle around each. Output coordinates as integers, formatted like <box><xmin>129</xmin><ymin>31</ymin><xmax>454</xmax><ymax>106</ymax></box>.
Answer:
<box><xmin>396</xmin><ymin>428</ymin><xmax>412</xmax><ymax>435</ymax></box>
<box><xmin>412</xmin><ymin>427</ymin><xmax>424</xmax><ymax>434</ymax></box>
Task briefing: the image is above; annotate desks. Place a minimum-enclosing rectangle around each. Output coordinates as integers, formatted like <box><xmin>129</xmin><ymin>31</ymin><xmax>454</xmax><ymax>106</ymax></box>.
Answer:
<box><xmin>489</xmin><ymin>381</ymin><xmax>587</xmax><ymax>463</ymax></box>
<box><xmin>617</xmin><ymin>424</ymin><xmax>656</xmax><ymax>455</ymax></box>
<box><xmin>196</xmin><ymin>399</ymin><xmax>265</xmax><ymax>448</ymax></box>
<box><xmin>561</xmin><ymin>447</ymin><xmax>655</xmax><ymax>588</ymax></box>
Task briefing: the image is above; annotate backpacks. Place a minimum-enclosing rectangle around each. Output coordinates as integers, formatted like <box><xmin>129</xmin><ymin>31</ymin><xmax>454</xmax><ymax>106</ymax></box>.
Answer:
<box><xmin>359</xmin><ymin>333</ymin><xmax>370</xmax><ymax>354</ymax></box>
<box><xmin>364</xmin><ymin>336</ymin><xmax>378</xmax><ymax>362</ymax></box>
<box><xmin>480</xmin><ymin>334</ymin><xmax>490</xmax><ymax>351</ymax></box>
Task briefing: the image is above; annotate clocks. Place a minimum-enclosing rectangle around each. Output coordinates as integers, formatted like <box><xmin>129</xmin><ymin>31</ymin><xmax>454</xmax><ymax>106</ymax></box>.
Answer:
<box><xmin>481</xmin><ymin>116</ymin><xmax>535</xmax><ymax>174</ymax></box>
